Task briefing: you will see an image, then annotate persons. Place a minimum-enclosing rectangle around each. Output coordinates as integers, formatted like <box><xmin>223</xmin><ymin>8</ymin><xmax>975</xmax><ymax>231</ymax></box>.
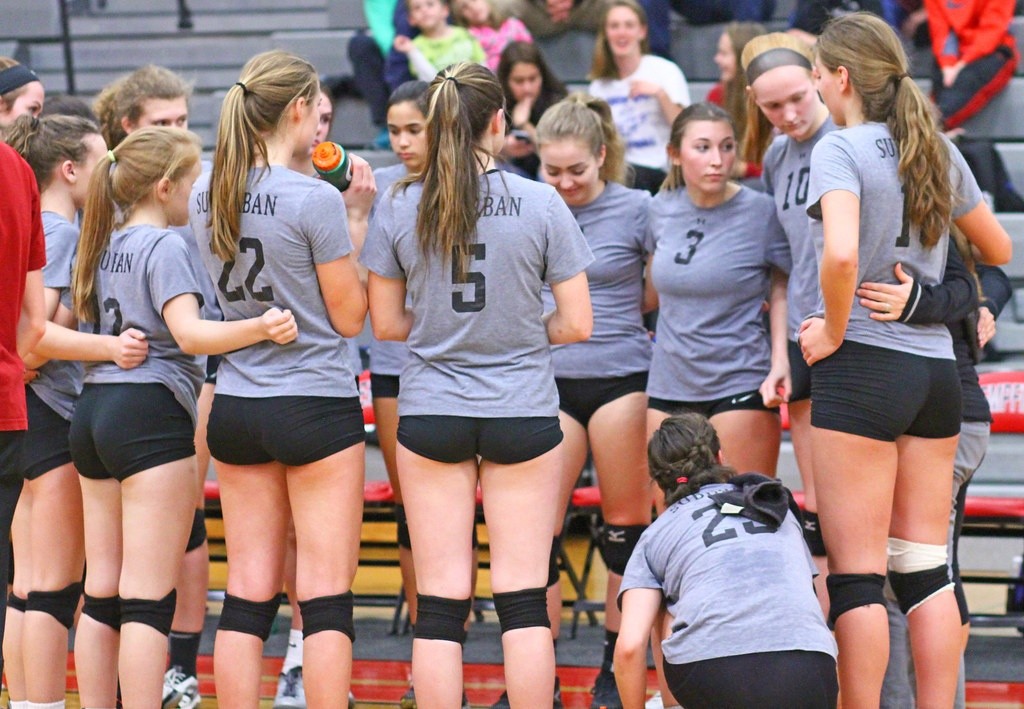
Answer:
<box><xmin>497</xmin><ymin>40</ymin><xmax>567</xmax><ymax>175</ymax></box>
<box><xmin>0</xmin><ymin>0</ymin><xmax>1018</xmax><ymax>709</ymax></box>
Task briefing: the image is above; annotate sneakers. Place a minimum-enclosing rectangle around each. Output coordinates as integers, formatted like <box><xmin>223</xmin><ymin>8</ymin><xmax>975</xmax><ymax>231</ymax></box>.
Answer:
<box><xmin>400</xmin><ymin>686</ymin><xmax>470</xmax><ymax>709</ymax></box>
<box><xmin>591</xmin><ymin>662</ymin><xmax>623</xmax><ymax>709</ymax></box>
<box><xmin>163</xmin><ymin>668</ymin><xmax>202</xmax><ymax>709</ymax></box>
<box><xmin>349</xmin><ymin>691</ymin><xmax>355</xmax><ymax>709</ymax></box>
<box><xmin>273</xmin><ymin>666</ymin><xmax>306</xmax><ymax>709</ymax></box>
<box><xmin>491</xmin><ymin>676</ymin><xmax>563</xmax><ymax>709</ymax></box>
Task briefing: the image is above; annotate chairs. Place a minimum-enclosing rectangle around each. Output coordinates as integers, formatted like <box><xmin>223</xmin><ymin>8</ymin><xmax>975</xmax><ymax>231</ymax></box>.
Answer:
<box><xmin>202</xmin><ymin>370</ymin><xmax>1024</xmax><ymax>640</ymax></box>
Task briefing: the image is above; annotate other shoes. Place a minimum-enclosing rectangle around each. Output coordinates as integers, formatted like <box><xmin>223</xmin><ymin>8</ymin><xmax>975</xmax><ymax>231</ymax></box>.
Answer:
<box><xmin>161</xmin><ymin>683</ymin><xmax>183</xmax><ymax>709</ymax></box>
<box><xmin>1006</xmin><ymin>583</ymin><xmax>1024</xmax><ymax>633</ymax></box>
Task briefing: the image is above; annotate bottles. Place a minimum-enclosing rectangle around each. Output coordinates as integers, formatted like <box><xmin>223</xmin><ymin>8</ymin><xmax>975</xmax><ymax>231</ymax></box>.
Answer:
<box><xmin>311</xmin><ymin>141</ymin><xmax>353</xmax><ymax>192</ymax></box>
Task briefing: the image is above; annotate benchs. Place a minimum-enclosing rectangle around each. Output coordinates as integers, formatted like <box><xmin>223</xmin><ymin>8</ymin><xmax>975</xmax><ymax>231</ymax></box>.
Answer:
<box><xmin>168</xmin><ymin>0</ymin><xmax>1024</xmax><ymax>590</ymax></box>
